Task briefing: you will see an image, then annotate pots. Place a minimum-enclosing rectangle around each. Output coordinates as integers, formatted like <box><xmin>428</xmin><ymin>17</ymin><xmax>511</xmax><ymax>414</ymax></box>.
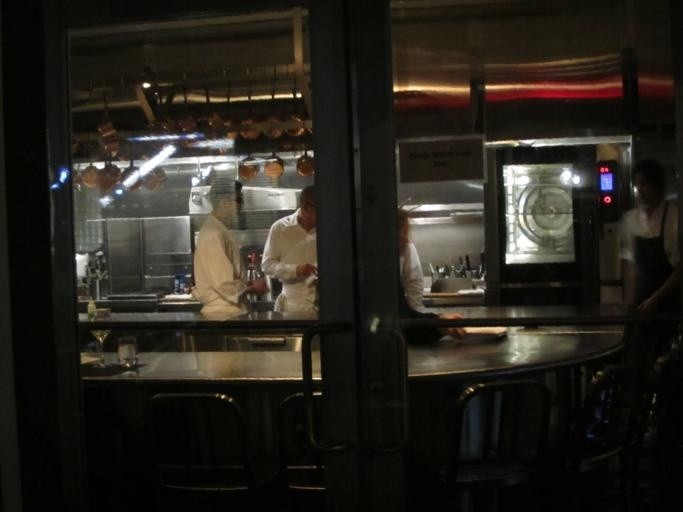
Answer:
<box><xmin>77</xmin><ymin>112</ymin><xmax>315</xmax><ymax>191</ymax></box>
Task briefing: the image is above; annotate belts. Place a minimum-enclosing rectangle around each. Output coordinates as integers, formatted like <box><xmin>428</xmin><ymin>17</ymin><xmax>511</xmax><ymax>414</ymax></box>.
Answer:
<box><xmin>87</xmin><ymin>308</ymin><xmax>112</xmax><ymax>371</ymax></box>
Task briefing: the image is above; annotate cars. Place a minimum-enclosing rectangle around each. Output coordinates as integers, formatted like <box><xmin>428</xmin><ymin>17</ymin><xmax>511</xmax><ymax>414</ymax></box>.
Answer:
<box><xmin>117</xmin><ymin>336</ymin><xmax>137</xmax><ymax>369</ymax></box>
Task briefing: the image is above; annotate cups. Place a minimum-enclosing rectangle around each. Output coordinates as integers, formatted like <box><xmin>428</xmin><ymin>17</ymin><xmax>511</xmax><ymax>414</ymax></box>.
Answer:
<box><xmin>229</xmin><ymin>196</ymin><xmax>242</xmax><ymax>204</ymax></box>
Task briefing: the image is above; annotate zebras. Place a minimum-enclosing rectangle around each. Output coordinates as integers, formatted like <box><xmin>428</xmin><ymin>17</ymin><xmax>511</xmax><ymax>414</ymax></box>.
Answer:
<box><xmin>480</xmin><ymin>134</ymin><xmax>635</xmax><ymax>306</ymax></box>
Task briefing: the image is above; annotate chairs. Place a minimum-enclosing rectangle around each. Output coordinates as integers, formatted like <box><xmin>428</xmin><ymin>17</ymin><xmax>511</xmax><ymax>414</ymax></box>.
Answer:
<box><xmin>136</xmin><ymin>337</ymin><xmax>683</xmax><ymax>510</ymax></box>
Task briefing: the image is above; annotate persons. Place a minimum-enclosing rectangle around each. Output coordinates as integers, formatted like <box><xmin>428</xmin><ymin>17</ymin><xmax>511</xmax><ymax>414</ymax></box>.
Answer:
<box><xmin>400</xmin><ymin>207</ymin><xmax>470</xmax><ymax>347</ymax></box>
<box><xmin>193</xmin><ymin>177</ymin><xmax>272</xmax><ymax>350</ymax></box>
<box><xmin>616</xmin><ymin>158</ymin><xmax>682</xmax><ymax>314</ymax></box>
<box><xmin>261</xmin><ymin>186</ymin><xmax>318</xmax><ymax>314</ymax></box>
